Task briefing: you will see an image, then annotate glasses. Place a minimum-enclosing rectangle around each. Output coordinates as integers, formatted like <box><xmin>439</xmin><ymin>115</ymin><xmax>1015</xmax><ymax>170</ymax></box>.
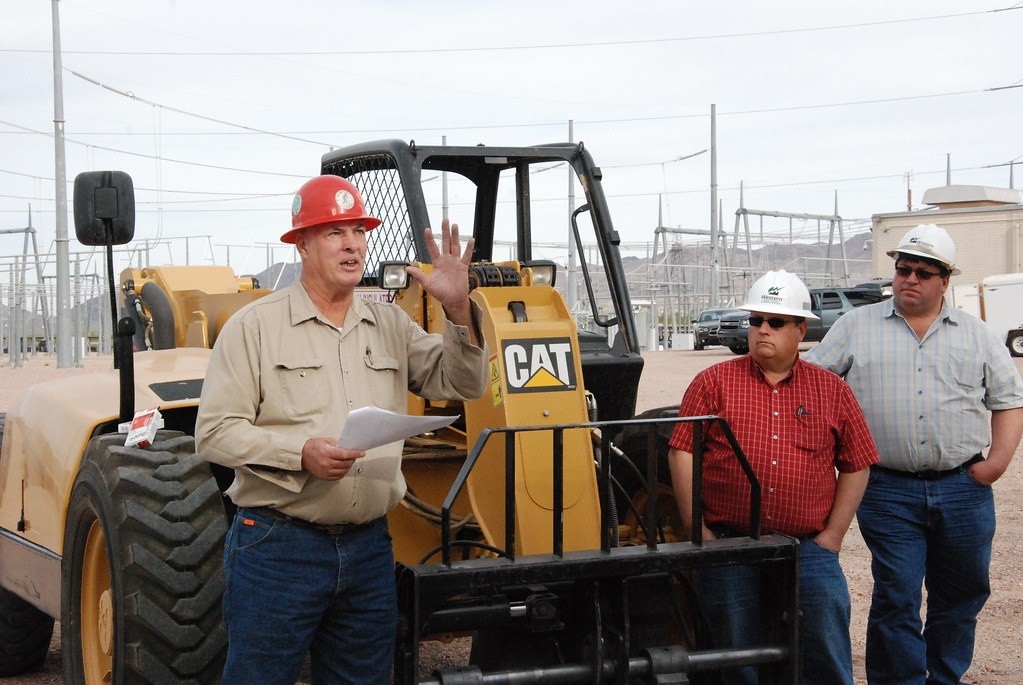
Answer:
<box><xmin>896</xmin><ymin>264</ymin><xmax>940</xmax><ymax>279</ymax></box>
<box><xmin>749</xmin><ymin>316</ymin><xmax>792</xmax><ymax>328</ymax></box>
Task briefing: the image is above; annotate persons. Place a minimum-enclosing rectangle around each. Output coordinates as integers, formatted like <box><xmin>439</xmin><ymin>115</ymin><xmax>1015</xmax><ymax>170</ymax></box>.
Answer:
<box><xmin>189</xmin><ymin>173</ymin><xmax>488</xmax><ymax>685</ymax></box>
<box><xmin>667</xmin><ymin>271</ymin><xmax>879</xmax><ymax>685</ymax></box>
<box><xmin>801</xmin><ymin>224</ymin><xmax>1023</xmax><ymax>685</ymax></box>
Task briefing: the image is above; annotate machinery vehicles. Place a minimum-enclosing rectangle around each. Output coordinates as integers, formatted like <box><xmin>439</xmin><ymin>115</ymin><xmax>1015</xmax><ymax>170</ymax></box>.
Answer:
<box><xmin>1</xmin><ymin>140</ymin><xmax>804</xmax><ymax>684</ymax></box>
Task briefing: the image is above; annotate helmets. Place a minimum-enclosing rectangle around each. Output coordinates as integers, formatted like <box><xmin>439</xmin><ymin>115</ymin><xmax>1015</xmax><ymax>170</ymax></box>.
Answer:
<box><xmin>737</xmin><ymin>272</ymin><xmax>824</xmax><ymax>322</ymax></box>
<box><xmin>277</xmin><ymin>174</ymin><xmax>382</xmax><ymax>241</ymax></box>
<box><xmin>887</xmin><ymin>223</ymin><xmax>960</xmax><ymax>279</ymax></box>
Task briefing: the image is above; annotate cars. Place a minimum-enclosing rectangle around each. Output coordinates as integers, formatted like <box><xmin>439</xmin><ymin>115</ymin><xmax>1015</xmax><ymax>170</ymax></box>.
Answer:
<box><xmin>691</xmin><ymin>307</ymin><xmax>738</xmax><ymax>350</ymax></box>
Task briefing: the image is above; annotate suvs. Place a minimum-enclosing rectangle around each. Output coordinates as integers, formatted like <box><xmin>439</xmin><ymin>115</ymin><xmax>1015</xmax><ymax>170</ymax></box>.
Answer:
<box><xmin>717</xmin><ymin>279</ymin><xmax>894</xmax><ymax>355</ymax></box>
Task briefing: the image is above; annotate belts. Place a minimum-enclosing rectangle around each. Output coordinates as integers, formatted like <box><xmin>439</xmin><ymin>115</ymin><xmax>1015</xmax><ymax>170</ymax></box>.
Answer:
<box><xmin>253</xmin><ymin>504</ymin><xmax>378</xmax><ymax>535</ymax></box>
<box><xmin>869</xmin><ymin>455</ymin><xmax>988</xmax><ymax>478</ymax></box>
